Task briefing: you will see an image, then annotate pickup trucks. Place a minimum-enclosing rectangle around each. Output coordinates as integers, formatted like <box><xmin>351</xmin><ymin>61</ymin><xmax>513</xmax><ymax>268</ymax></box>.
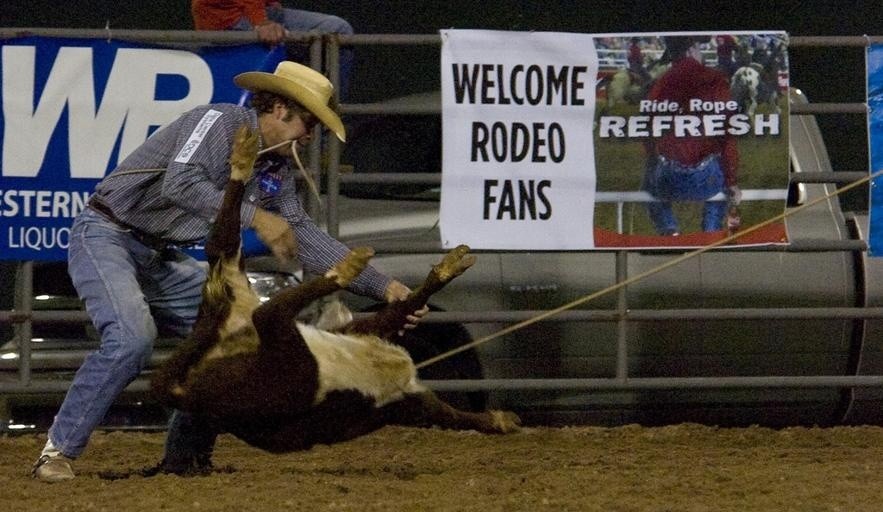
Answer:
<box><xmin>242</xmin><ymin>78</ymin><xmax>882</xmax><ymax>441</ymax></box>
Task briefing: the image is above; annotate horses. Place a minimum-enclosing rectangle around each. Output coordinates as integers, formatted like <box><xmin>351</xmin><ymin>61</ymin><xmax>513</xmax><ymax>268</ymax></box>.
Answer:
<box><xmin>729</xmin><ymin>52</ymin><xmax>786</xmax><ymax>117</ymax></box>
<box><xmin>596</xmin><ymin>57</ymin><xmax>667</xmax><ymax>115</ymax></box>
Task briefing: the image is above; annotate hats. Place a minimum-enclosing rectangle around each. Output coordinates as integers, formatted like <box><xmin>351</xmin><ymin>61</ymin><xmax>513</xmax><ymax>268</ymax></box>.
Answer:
<box><xmin>660</xmin><ymin>36</ymin><xmax>711</xmax><ymax>65</ymax></box>
<box><xmin>233</xmin><ymin>61</ymin><xmax>346</xmax><ymax>142</ymax></box>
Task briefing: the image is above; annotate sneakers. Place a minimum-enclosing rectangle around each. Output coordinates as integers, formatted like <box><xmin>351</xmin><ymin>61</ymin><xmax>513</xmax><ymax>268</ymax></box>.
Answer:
<box><xmin>32</xmin><ymin>455</ymin><xmax>75</xmax><ymax>481</ymax></box>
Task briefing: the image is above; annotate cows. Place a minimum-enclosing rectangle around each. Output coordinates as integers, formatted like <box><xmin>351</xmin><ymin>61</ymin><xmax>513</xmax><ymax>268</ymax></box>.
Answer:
<box><xmin>149</xmin><ymin>122</ymin><xmax>523</xmax><ymax>454</ymax></box>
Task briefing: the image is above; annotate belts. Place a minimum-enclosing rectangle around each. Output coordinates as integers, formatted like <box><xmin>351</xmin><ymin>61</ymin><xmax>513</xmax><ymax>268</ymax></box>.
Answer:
<box><xmin>87</xmin><ymin>198</ymin><xmax>154</xmax><ymax>245</ymax></box>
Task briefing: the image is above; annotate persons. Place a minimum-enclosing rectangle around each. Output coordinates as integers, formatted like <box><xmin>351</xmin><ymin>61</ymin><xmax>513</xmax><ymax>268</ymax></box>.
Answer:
<box><xmin>190</xmin><ymin>1</ymin><xmax>353</xmax><ymax>103</ymax></box>
<box><xmin>595</xmin><ymin>34</ymin><xmax>788</xmax><ymax>239</ymax></box>
<box><xmin>29</xmin><ymin>61</ymin><xmax>430</xmax><ymax>482</ymax></box>
